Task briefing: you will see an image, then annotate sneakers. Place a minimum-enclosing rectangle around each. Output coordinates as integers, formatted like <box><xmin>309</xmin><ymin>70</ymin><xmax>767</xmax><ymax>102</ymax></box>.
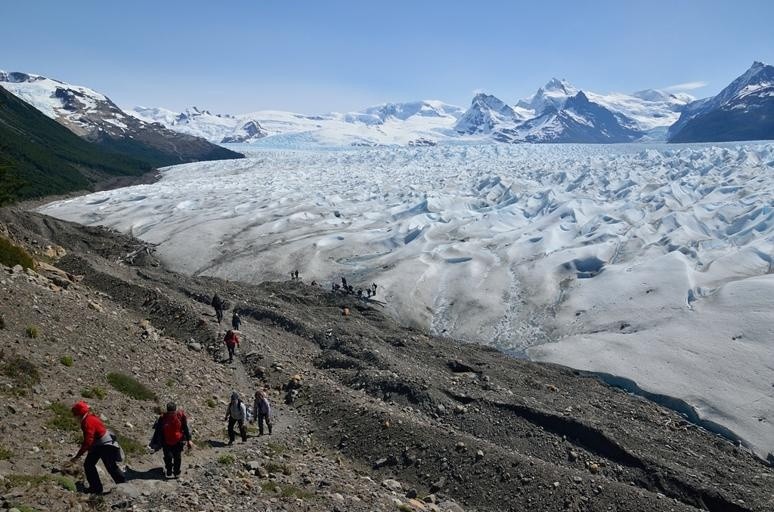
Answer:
<box><xmin>228</xmin><ymin>438</ymin><xmax>235</xmax><ymax>446</ymax></box>
<box><xmin>84</xmin><ymin>484</ymin><xmax>104</xmax><ymax>494</ymax></box>
<box><xmin>116</xmin><ymin>474</ymin><xmax>127</xmax><ymax>484</ymax></box>
<box><xmin>166</xmin><ymin>469</ymin><xmax>181</xmax><ymax>477</ymax></box>
<box><xmin>242</xmin><ymin>436</ymin><xmax>247</xmax><ymax>442</ymax></box>
<box><xmin>269</xmin><ymin>428</ymin><xmax>273</xmax><ymax>435</ymax></box>
<box><xmin>257</xmin><ymin>432</ymin><xmax>264</xmax><ymax>437</ymax></box>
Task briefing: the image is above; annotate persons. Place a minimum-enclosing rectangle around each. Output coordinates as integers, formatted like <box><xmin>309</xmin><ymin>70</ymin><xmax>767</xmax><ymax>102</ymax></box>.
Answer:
<box><xmin>223</xmin><ymin>391</ymin><xmax>248</xmax><ymax>446</ymax></box>
<box><xmin>231</xmin><ymin>311</ymin><xmax>242</xmax><ymax>331</ymax></box>
<box><xmin>69</xmin><ymin>400</ymin><xmax>128</xmax><ymax>495</ymax></box>
<box><xmin>290</xmin><ymin>269</ymin><xmax>377</xmax><ymax>302</ymax></box>
<box><xmin>211</xmin><ymin>292</ymin><xmax>220</xmax><ymax>308</ymax></box>
<box><xmin>223</xmin><ymin>329</ymin><xmax>240</xmax><ymax>364</ymax></box>
<box><xmin>252</xmin><ymin>389</ymin><xmax>273</xmax><ymax>437</ymax></box>
<box><xmin>215</xmin><ymin>299</ymin><xmax>229</xmax><ymax>324</ymax></box>
<box><xmin>153</xmin><ymin>400</ymin><xmax>193</xmax><ymax>477</ymax></box>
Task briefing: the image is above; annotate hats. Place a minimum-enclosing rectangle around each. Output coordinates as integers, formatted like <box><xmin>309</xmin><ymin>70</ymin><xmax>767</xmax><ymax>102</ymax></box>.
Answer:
<box><xmin>70</xmin><ymin>401</ymin><xmax>88</xmax><ymax>416</ymax></box>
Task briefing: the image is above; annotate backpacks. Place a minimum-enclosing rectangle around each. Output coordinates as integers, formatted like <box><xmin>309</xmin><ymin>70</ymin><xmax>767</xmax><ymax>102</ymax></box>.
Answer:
<box><xmin>160</xmin><ymin>410</ymin><xmax>185</xmax><ymax>449</ymax></box>
<box><xmin>229</xmin><ymin>399</ymin><xmax>247</xmax><ymax>415</ymax></box>
